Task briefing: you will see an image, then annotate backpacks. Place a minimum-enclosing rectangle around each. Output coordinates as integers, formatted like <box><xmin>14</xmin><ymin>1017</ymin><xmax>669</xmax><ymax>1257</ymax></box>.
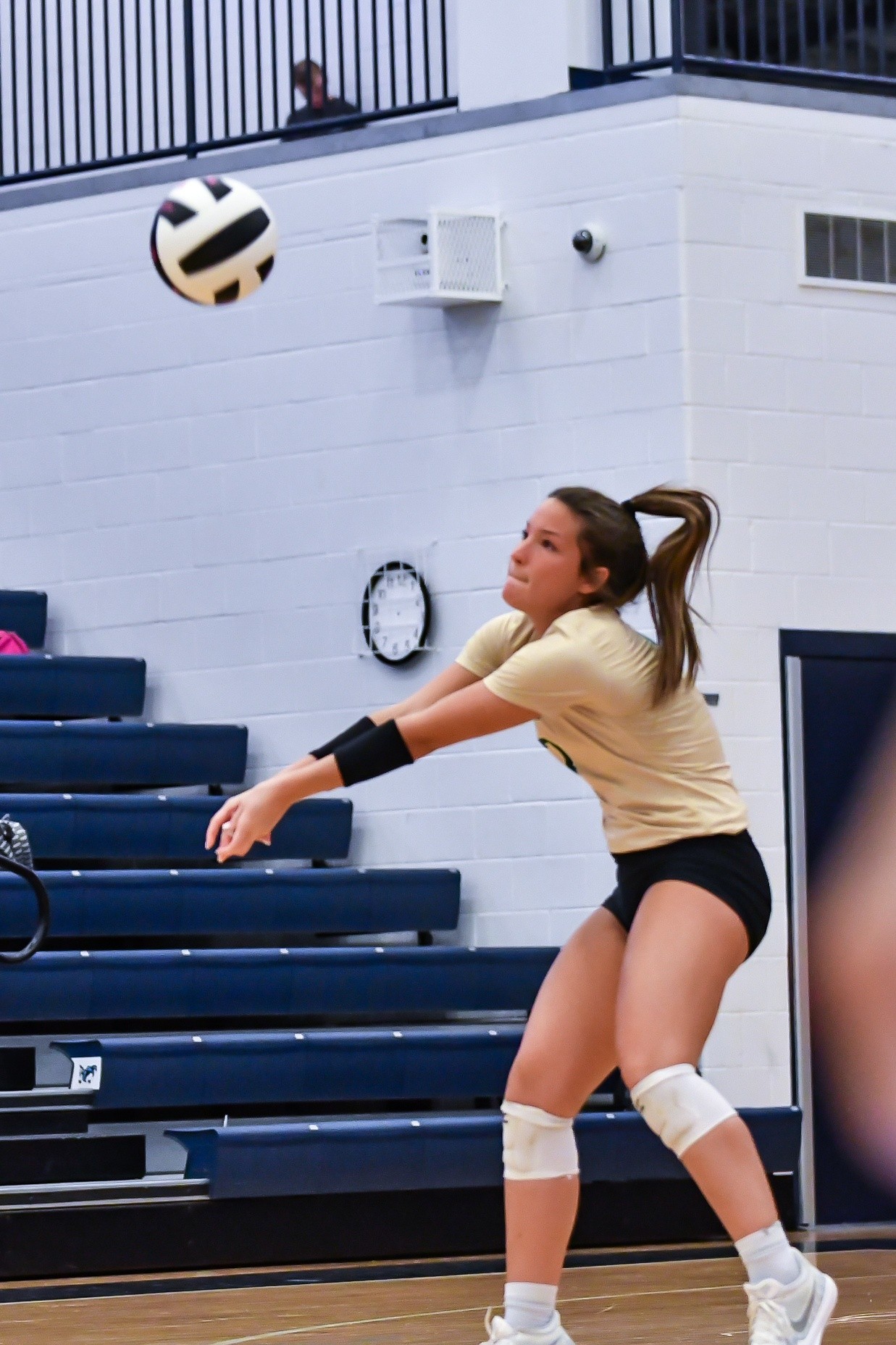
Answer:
<box><xmin>0</xmin><ymin>812</ymin><xmax>34</xmax><ymax>870</ymax></box>
<box><xmin>0</xmin><ymin>629</ymin><xmax>29</xmax><ymax>655</ymax></box>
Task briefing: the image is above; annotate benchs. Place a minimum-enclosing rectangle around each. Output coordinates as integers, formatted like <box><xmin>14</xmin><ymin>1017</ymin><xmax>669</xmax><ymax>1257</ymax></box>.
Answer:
<box><xmin>0</xmin><ymin>590</ymin><xmax>801</xmax><ymax>1200</ymax></box>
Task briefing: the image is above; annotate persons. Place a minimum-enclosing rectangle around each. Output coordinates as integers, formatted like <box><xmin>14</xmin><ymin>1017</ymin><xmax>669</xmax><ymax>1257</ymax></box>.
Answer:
<box><xmin>282</xmin><ymin>52</ymin><xmax>368</xmax><ymax>140</ymax></box>
<box><xmin>204</xmin><ymin>484</ymin><xmax>841</xmax><ymax>1345</ymax></box>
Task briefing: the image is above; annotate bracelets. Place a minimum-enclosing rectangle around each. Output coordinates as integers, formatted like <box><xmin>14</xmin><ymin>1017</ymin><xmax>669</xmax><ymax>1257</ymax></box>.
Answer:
<box><xmin>334</xmin><ymin>719</ymin><xmax>415</xmax><ymax>787</ymax></box>
<box><xmin>307</xmin><ymin>715</ymin><xmax>378</xmax><ymax>760</ymax></box>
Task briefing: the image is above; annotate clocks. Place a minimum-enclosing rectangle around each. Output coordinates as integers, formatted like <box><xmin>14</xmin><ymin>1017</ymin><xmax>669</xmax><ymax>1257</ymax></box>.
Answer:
<box><xmin>361</xmin><ymin>562</ymin><xmax>435</xmax><ymax>668</ymax></box>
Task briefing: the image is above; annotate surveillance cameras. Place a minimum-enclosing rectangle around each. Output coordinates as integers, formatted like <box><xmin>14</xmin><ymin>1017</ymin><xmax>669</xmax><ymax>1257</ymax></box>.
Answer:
<box><xmin>572</xmin><ymin>224</ymin><xmax>603</xmax><ymax>261</ymax></box>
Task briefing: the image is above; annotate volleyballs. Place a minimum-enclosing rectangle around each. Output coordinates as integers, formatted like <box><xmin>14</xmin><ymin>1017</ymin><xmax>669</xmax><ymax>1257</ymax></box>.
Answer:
<box><xmin>150</xmin><ymin>174</ymin><xmax>277</xmax><ymax>306</ymax></box>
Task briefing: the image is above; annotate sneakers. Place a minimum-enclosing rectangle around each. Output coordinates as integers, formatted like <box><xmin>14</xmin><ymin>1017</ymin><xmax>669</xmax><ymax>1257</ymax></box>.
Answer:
<box><xmin>479</xmin><ymin>1307</ymin><xmax>576</xmax><ymax>1345</ymax></box>
<box><xmin>743</xmin><ymin>1247</ymin><xmax>839</xmax><ymax>1345</ymax></box>
